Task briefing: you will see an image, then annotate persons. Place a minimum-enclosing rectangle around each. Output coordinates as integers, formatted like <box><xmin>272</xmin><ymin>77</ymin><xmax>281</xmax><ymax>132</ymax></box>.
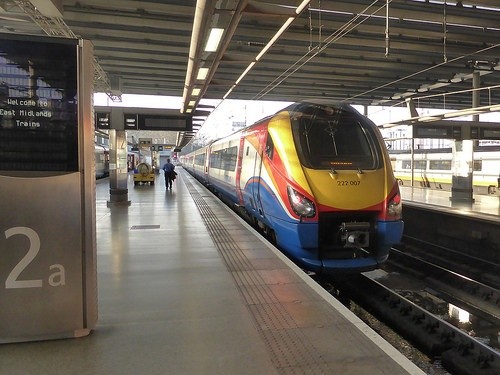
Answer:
<box><xmin>163</xmin><ymin>158</ymin><xmax>174</xmax><ymax>190</ymax></box>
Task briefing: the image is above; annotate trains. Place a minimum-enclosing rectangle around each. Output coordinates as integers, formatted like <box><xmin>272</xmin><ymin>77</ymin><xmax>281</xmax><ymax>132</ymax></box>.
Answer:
<box><xmin>389</xmin><ymin>147</ymin><xmax>500</xmax><ymax>194</ymax></box>
<box><xmin>179</xmin><ymin>98</ymin><xmax>405</xmax><ymax>274</ymax></box>
<box><xmin>95</xmin><ymin>130</ymin><xmax>132</xmax><ymax>181</ymax></box>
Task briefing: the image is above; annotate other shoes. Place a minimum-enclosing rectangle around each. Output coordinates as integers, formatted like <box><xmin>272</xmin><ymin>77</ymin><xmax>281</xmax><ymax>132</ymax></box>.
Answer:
<box><xmin>166</xmin><ymin>187</ymin><xmax>168</xmax><ymax>189</ymax></box>
<box><xmin>170</xmin><ymin>188</ymin><xmax>172</xmax><ymax>190</ymax></box>
